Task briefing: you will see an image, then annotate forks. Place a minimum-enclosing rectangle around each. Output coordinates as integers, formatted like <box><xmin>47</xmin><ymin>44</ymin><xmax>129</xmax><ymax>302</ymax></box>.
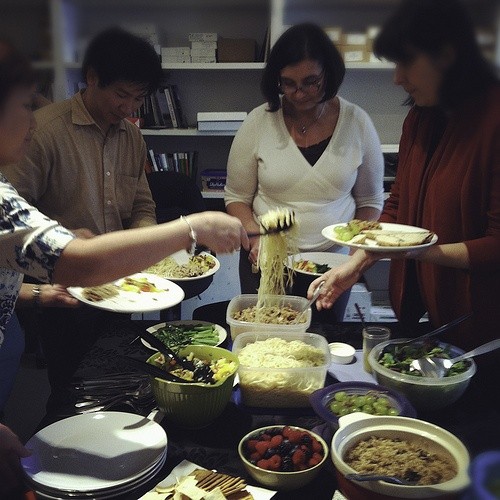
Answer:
<box><xmin>73</xmin><ymin>377</ymin><xmax>158</xmax><ymax>415</ymax></box>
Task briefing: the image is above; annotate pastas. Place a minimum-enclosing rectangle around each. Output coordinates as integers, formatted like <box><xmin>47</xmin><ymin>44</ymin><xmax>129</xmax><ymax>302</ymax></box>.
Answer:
<box><xmin>145</xmin><ymin>254</ymin><xmax>199</xmax><ymax>278</ymax></box>
<box><xmin>237</xmin><ymin>208</ymin><xmax>326</xmax><ymax>409</ymax></box>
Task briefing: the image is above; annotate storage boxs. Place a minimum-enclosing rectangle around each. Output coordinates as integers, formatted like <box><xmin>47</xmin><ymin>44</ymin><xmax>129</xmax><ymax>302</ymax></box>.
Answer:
<box><xmin>160</xmin><ymin>32</ymin><xmax>218</xmax><ymax>63</ymax></box>
<box><xmin>324</xmin><ymin>24</ymin><xmax>380</xmax><ymax>63</ymax></box>
<box><xmin>343</xmin><ymin>283</ymin><xmax>372</xmax><ymax>322</ymax></box>
<box><xmin>199</xmin><ymin>169</ymin><xmax>228</xmax><ymax>192</ymax></box>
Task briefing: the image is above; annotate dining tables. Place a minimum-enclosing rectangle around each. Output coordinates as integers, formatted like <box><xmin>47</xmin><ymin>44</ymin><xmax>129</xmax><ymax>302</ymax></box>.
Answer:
<box><xmin>0</xmin><ymin>320</ymin><xmax>500</xmax><ymax>500</ymax></box>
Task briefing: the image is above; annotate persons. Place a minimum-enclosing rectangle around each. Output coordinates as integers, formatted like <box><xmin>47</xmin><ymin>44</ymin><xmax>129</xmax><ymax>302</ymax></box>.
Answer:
<box><xmin>3</xmin><ymin>27</ymin><xmax>163</xmax><ymax>411</ymax></box>
<box><xmin>0</xmin><ymin>47</ymin><xmax>250</xmax><ymax>463</ymax></box>
<box><xmin>309</xmin><ymin>0</ymin><xmax>500</xmax><ymax>332</ymax></box>
<box><xmin>224</xmin><ymin>23</ymin><xmax>385</xmax><ymax>322</ymax></box>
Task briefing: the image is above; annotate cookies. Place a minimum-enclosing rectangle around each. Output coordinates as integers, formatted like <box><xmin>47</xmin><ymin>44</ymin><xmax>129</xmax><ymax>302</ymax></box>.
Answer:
<box><xmin>174</xmin><ymin>468</ymin><xmax>254</xmax><ymax>500</ymax></box>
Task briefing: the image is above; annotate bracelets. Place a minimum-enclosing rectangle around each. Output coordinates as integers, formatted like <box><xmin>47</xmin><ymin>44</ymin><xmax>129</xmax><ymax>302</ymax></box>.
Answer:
<box><xmin>33</xmin><ymin>284</ymin><xmax>40</xmax><ymax>304</ymax></box>
<box><xmin>180</xmin><ymin>216</ymin><xmax>196</xmax><ymax>253</ymax></box>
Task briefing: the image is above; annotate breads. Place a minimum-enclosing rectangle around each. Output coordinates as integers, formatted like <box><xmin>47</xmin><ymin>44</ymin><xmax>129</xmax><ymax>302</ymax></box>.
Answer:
<box><xmin>376</xmin><ymin>231</ymin><xmax>427</xmax><ymax>246</ymax></box>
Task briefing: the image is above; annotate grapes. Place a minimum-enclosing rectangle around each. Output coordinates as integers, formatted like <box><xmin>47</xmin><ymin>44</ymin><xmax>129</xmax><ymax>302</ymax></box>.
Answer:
<box><xmin>331</xmin><ymin>391</ymin><xmax>400</xmax><ymax>416</ymax></box>
<box><xmin>333</xmin><ymin>222</ymin><xmax>362</xmax><ymax>241</ymax></box>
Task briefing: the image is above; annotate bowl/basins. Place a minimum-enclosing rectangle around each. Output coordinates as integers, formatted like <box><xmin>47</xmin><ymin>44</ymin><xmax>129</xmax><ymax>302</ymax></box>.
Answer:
<box><xmin>368</xmin><ymin>338</ymin><xmax>477</xmax><ymax>411</ymax></box>
<box><xmin>238</xmin><ymin>425</ymin><xmax>329</xmax><ymax>493</ymax></box>
<box><xmin>231</xmin><ymin>332</ymin><xmax>330</xmax><ymax>409</ymax></box>
<box><xmin>143</xmin><ymin>345</ymin><xmax>239</xmax><ymax>430</ymax></box>
<box><xmin>310</xmin><ymin>381</ymin><xmax>417</xmax><ymax>430</ymax></box>
<box><xmin>225</xmin><ymin>293</ymin><xmax>312</xmax><ymax>343</ymax></box>
<box><xmin>330</xmin><ymin>412</ymin><xmax>472</xmax><ymax>500</ymax></box>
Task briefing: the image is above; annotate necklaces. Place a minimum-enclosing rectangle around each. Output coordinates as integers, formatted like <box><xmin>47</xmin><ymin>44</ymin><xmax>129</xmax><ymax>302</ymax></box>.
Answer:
<box><xmin>300</xmin><ymin>103</ymin><xmax>326</xmax><ymax>133</ymax></box>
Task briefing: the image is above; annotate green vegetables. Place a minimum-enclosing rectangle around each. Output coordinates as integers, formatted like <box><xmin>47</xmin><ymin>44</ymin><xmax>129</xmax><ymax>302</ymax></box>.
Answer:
<box><xmin>375</xmin><ymin>346</ymin><xmax>466</xmax><ymax>412</ymax></box>
<box><xmin>147</xmin><ymin>322</ymin><xmax>221</xmax><ymax>348</ymax></box>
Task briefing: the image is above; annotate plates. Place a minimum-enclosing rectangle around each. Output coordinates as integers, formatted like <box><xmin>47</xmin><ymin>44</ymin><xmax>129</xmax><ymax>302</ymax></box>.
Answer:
<box><xmin>136</xmin><ymin>458</ymin><xmax>278</xmax><ymax>500</ymax></box>
<box><xmin>66</xmin><ymin>273</ymin><xmax>186</xmax><ymax>313</ymax></box>
<box><xmin>139</xmin><ymin>320</ymin><xmax>228</xmax><ymax>353</ymax></box>
<box><xmin>283</xmin><ymin>251</ymin><xmax>352</xmax><ymax>276</ymax></box>
<box><xmin>141</xmin><ymin>248</ymin><xmax>220</xmax><ymax>282</ymax></box>
<box><xmin>321</xmin><ymin>222</ymin><xmax>440</xmax><ymax>253</ymax></box>
<box><xmin>20</xmin><ymin>410</ymin><xmax>167</xmax><ymax>500</ymax></box>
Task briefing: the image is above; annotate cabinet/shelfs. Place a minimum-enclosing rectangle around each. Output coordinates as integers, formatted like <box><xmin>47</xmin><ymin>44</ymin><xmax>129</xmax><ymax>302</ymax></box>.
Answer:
<box><xmin>33</xmin><ymin>0</ymin><xmax>500</xmax><ymax>198</ymax></box>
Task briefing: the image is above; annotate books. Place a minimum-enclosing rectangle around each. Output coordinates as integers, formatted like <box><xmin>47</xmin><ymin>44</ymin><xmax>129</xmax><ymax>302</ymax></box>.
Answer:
<box><xmin>147</xmin><ymin>147</ymin><xmax>197</xmax><ymax>179</ymax></box>
<box><xmin>197</xmin><ymin>111</ymin><xmax>248</xmax><ymax>132</ymax></box>
<box><xmin>74</xmin><ymin>81</ymin><xmax>181</xmax><ymax>129</ymax></box>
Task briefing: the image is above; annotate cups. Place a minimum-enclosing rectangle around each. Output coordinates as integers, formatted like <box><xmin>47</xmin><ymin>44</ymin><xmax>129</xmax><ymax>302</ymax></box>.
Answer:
<box><xmin>363</xmin><ymin>326</ymin><xmax>391</xmax><ymax>373</ymax></box>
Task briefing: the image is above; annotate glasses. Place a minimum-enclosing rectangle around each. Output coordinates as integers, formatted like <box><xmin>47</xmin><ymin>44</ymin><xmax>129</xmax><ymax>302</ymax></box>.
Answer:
<box><xmin>276</xmin><ymin>71</ymin><xmax>326</xmax><ymax>96</ymax></box>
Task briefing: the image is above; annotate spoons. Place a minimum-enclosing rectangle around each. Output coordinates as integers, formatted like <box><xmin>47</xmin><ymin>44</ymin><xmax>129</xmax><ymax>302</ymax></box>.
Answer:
<box><xmin>408</xmin><ymin>338</ymin><xmax>500</xmax><ymax>378</ymax></box>
